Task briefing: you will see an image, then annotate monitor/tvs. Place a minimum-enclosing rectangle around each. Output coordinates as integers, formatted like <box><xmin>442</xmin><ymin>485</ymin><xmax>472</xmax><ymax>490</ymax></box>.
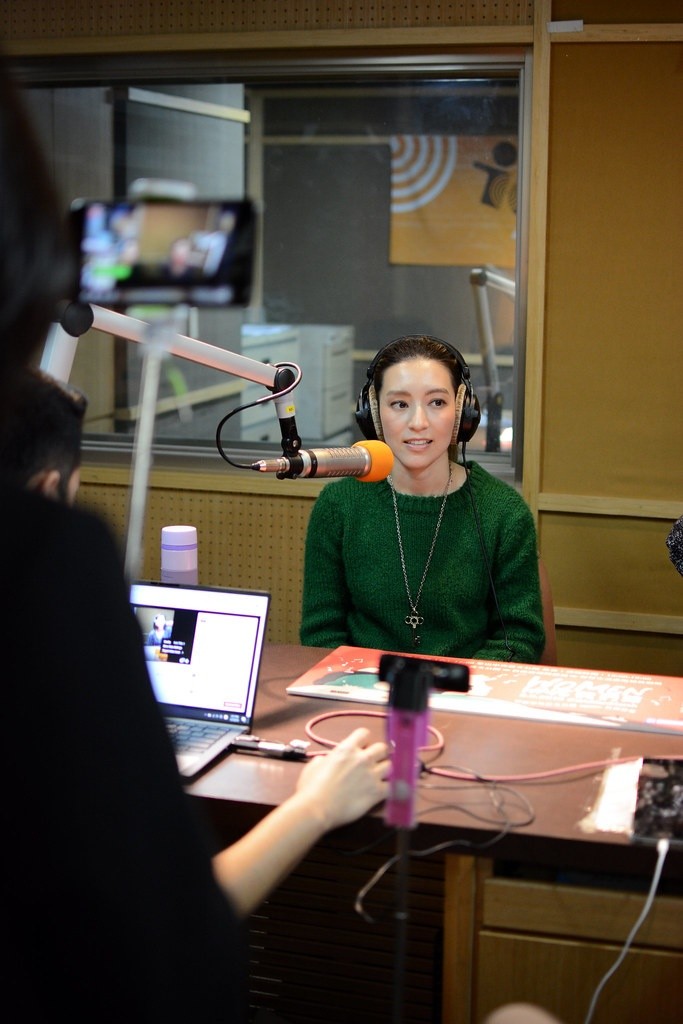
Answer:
<box><xmin>71</xmin><ymin>197</ymin><xmax>258</xmax><ymax>308</ymax></box>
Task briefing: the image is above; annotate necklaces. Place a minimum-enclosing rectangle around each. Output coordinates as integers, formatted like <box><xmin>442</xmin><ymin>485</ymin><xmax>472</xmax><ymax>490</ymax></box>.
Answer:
<box><xmin>386</xmin><ymin>459</ymin><xmax>453</xmax><ymax>649</ymax></box>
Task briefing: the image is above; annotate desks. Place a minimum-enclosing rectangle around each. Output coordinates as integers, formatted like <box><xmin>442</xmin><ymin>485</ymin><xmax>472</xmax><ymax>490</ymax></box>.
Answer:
<box><xmin>184</xmin><ymin>639</ymin><xmax>683</xmax><ymax>1024</ymax></box>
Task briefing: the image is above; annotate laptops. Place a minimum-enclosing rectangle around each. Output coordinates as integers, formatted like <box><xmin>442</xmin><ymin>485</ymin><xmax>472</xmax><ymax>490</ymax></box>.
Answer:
<box><xmin>130</xmin><ymin>581</ymin><xmax>272</xmax><ymax>782</ymax></box>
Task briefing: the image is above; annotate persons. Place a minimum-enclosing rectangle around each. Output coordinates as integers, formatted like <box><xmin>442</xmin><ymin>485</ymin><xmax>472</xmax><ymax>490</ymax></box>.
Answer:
<box><xmin>8</xmin><ymin>371</ymin><xmax>393</xmax><ymax>938</ymax></box>
<box><xmin>154</xmin><ymin>235</ymin><xmax>208</xmax><ymax>284</ymax></box>
<box><xmin>110</xmin><ymin>239</ymin><xmax>158</xmax><ymax>290</ymax></box>
<box><xmin>299</xmin><ymin>335</ymin><xmax>545</xmax><ymax>664</ymax></box>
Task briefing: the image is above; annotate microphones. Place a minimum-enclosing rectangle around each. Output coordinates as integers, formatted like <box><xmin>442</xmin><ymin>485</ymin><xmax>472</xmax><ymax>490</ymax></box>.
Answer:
<box><xmin>252</xmin><ymin>439</ymin><xmax>394</xmax><ymax>482</ymax></box>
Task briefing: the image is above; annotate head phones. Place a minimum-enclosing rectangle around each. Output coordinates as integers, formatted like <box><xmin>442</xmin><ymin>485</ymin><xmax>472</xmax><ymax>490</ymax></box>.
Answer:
<box><xmin>355</xmin><ymin>334</ymin><xmax>481</xmax><ymax>443</ymax></box>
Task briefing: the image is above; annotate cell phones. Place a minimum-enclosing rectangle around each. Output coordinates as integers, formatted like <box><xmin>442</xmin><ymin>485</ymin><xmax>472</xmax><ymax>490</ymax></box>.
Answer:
<box><xmin>633</xmin><ymin>758</ymin><xmax>683</xmax><ymax>846</ymax></box>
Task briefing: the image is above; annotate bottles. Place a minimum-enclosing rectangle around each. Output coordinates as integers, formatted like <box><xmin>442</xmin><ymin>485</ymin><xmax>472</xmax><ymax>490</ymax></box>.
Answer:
<box><xmin>161</xmin><ymin>526</ymin><xmax>198</xmax><ymax>585</ymax></box>
